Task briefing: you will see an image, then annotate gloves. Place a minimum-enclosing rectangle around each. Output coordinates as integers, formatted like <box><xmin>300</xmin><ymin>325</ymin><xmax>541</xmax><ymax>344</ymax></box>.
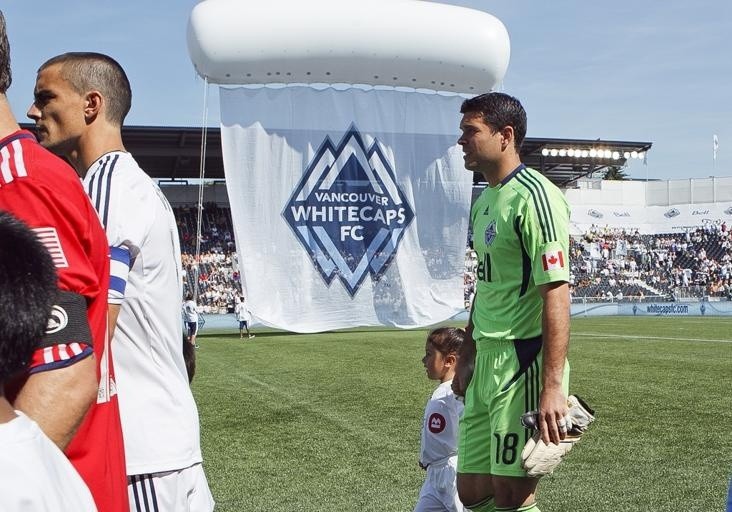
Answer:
<box><xmin>517</xmin><ymin>392</ymin><xmax>597</xmax><ymax>480</ymax></box>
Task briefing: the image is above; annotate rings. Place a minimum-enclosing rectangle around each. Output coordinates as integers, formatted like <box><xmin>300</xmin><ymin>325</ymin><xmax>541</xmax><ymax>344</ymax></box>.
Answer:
<box><xmin>559</xmin><ymin>426</ymin><xmax>568</xmax><ymax>434</ymax></box>
<box><xmin>556</xmin><ymin>418</ymin><xmax>567</xmax><ymax>427</ymax></box>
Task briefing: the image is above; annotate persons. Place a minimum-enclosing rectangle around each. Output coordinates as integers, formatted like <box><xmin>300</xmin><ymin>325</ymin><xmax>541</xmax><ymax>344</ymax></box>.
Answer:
<box><xmin>451</xmin><ymin>89</ymin><xmax>576</xmax><ymax>512</ymax></box>
<box><xmin>0</xmin><ymin>8</ymin><xmax>139</xmax><ymax>512</ymax></box>
<box><xmin>170</xmin><ymin>198</ymin><xmax>732</xmax><ymax>316</ymax></box>
<box><xmin>182</xmin><ymin>292</ymin><xmax>203</xmax><ymax>349</ymax></box>
<box><xmin>181</xmin><ymin>297</ymin><xmax>189</xmax><ymax>331</ymax></box>
<box><xmin>234</xmin><ymin>297</ymin><xmax>255</xmax><ymax>340</ymax></box>
<box><xmin>181</xmin><ymin>330</ymin><xmax>197</xmax><ymax>387</ymax></box>
<box><xmin>409</xmin><ymin>325</ymin><xmax>474</xmax><ymax>512</ymax></box>
<box><xmin>25</xmin><ymin>49</ymin><xmax>219</xmax><ymax>512</ymax></box>
<box><xmin>1</xmin><ymin>208</ymin><xmax>100</xmax><ymax>512</ymax></box>
